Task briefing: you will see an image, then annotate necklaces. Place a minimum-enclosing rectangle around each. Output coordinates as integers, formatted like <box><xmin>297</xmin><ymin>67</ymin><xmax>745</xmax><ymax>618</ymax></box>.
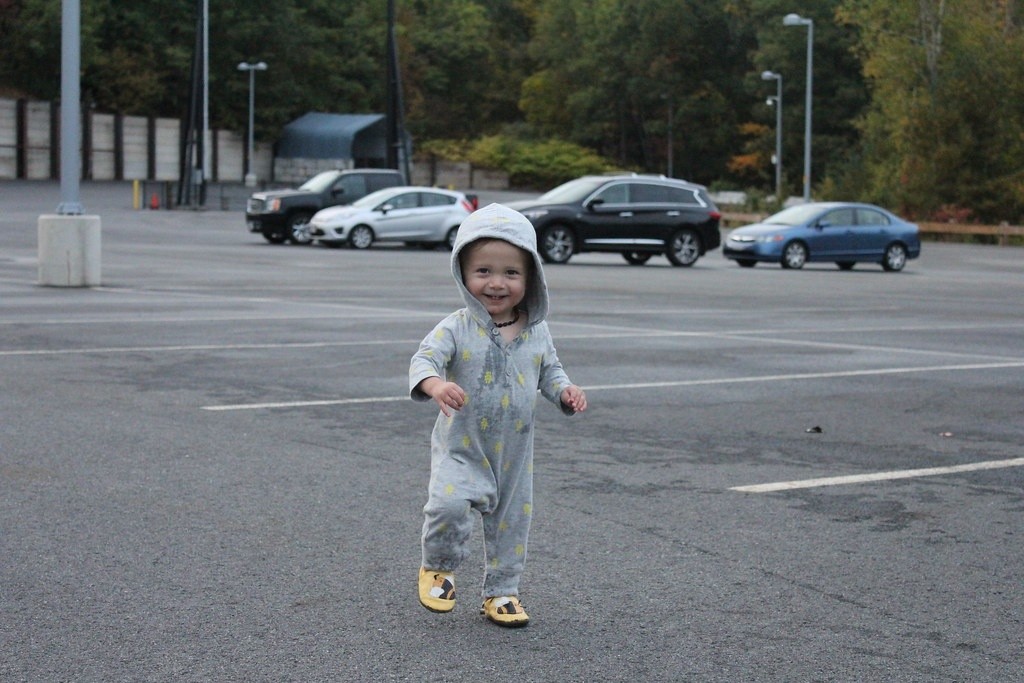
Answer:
<box><xmin>487</xmin><ymin>308</ymin><xmax>520</xmax><ymax>329</ymax></box>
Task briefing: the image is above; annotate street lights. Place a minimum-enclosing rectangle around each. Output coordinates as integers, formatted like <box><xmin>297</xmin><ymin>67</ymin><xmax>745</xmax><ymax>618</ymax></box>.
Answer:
<box><xmin>236</xmin><ymin>61</ymin><xmax>267</xmax><ymax>186</ymax></box>
<box><xmin>762</xmin><ymin>70</ymin><xmax>783</xmax><ymax>195</ymax></box>
<box><xmin>782</xmin><ymin>12</ymin><xmax>813</xmax><ymax>200</ymax></box>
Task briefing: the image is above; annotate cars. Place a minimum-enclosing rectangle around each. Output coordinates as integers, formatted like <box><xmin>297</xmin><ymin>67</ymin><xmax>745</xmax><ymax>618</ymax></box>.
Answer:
<box><xmin>309</xmin><ymin>185</ymin><xmax>475</xmax><ymax>251</ymax></box>
<box><xmin>724</xmin><ymin>201</ymin><xmax>921</xmax><ymax>271</ymax></box>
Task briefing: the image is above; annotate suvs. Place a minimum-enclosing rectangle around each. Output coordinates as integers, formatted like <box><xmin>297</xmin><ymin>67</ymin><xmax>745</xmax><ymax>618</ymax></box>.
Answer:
<box><xmin>503</xmin><ymin>172</ymin><xmax>723</xmax><ymax>268</ymax></box>
<box><xmin>245</xmin><ymin>167</ymin><xmax>406</xmax><ymax>245</ymax></box>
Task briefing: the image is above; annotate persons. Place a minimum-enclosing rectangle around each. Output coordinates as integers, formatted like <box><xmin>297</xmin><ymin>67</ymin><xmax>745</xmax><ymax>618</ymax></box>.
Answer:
<box><xmin>408</xmin><ymin>203</ymin><xmax>587</xmax><ymax>629</ymax></box>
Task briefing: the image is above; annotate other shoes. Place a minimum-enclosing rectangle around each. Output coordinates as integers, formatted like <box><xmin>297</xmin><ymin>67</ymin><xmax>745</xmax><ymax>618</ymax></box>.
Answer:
<box><xmin>480</xmin><ymin>596</ymin><xmax>530</xmax><ymax>628</ymax></box>
<box><xmin>418</xmin><ymin>565</ymin><xmax>458</xmax><ymax>613</ymax></box>
